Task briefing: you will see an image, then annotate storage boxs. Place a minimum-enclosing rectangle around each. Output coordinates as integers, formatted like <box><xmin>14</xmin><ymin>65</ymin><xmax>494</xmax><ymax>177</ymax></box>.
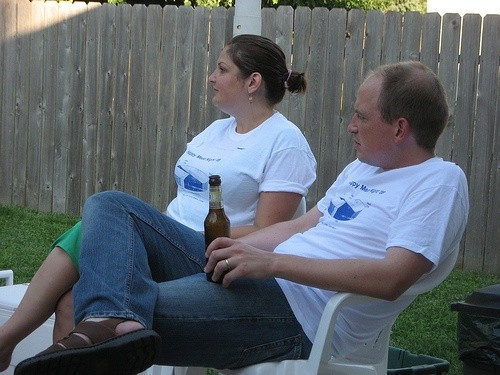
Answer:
<box><xmin>384</xmin><ymin>346</ymin><xmax>449</xmax><ymax>375</ymax></box>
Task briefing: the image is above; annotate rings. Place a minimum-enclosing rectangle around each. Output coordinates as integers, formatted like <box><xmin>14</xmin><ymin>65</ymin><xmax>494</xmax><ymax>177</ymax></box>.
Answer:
<box><xmin>225</xmin><ymin>258</ymin><xmax>231</xmax><ymax>269</ymax></box>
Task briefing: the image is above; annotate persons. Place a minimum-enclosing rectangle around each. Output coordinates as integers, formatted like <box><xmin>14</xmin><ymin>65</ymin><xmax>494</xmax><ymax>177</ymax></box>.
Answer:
<box><xmin>8</xmin><ymin>61</ymin><xmax>470</xmax><ymax>375</ymax></box>
<box><xmin>0</xmin><ymin>35</ymin><xmax>318</xmax><ymax>371</ymax></box>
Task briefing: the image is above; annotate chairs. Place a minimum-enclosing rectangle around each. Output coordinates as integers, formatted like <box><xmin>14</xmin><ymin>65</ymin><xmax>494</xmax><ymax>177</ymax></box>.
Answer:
<box><xmin>174</xmin><ymin>243</ymin><xmax>461</xmax><ymax>375</ymax></box>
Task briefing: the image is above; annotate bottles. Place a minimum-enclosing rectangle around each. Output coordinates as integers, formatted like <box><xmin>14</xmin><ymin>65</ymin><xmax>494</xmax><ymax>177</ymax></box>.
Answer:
<box><xmin>204</xmin><ymin>175</ymin><xmax>230</xmax><ymax>283</ymax></box>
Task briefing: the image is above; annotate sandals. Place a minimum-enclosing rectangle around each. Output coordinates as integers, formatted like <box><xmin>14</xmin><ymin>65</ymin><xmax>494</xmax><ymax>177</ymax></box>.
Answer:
<box><xmin>14</xmin><ymin>318</ymin><xmax>162</xmax><ymax>375</ymax></box>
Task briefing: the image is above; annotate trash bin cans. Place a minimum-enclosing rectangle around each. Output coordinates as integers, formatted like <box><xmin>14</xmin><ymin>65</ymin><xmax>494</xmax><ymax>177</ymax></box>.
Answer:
<box><xmin>387</xmin><ymin>344</ymin><xmax>450</xmax><ymax>374</ymax></box>
<box><xmin>451</xmin><ymin>283</ymin><xmax>500</xmax><ymax>375</ymax></box>
